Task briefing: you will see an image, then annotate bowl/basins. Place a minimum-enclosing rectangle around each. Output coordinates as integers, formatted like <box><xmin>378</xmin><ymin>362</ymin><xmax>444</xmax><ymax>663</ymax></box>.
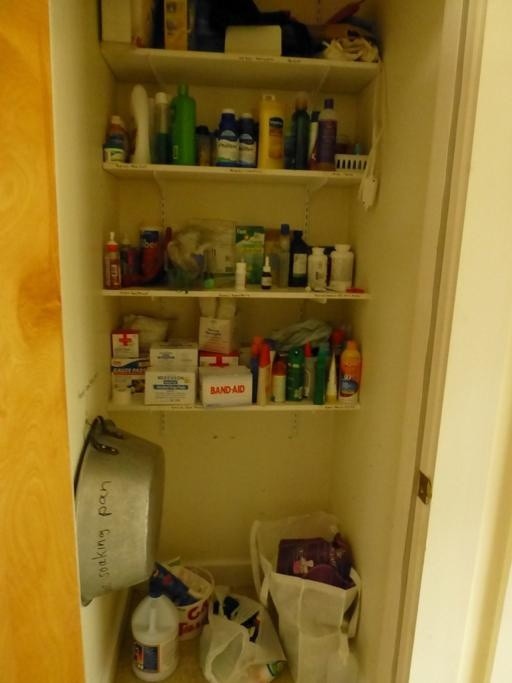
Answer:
<box><xmin>75</xmin><ymin>419</ymin><xmax>166</xmax><ymax>606</ymax></box>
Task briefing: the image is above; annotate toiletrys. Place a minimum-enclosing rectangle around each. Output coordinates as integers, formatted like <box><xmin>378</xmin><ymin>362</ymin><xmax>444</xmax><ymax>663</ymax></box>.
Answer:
<box><xmin>256</xmin><ymin>343</ymin><xmax>272</xmax><ymax>404</ymax></box>
<box><xmin>249</xmin><ymin>336</ymin><xmax>265</xmax><ymax>403</ymax></box>
<box><xmin>314</xmin><ymin>342</ymin><xmax>330</xmax><ymax>404</ymax></box>
<box><xmin>307</xmin><ymin>110</ymin><xmax>320</xmax><ymax>168</ymax></box>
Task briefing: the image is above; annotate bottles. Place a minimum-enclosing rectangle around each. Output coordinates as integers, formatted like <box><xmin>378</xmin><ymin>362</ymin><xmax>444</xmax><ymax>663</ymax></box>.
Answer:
<box><xmin>290</xmin><ymin>230</ymin><xmax>308</xmax><ymax>287</ymax></box>
<box><xmin>104</xmin><ymin>232</ymin><xmax>121</xmax><ymax>289</ymax></box>
<box><xmin>121</xmin><ymin>233</ymin><xmax>130</xmax><ymax>281</ymax></box>
<box><xmin>235</xmin><ymin>262</ymin><xmax>247</xmax><ymax>290</ymax></box>
<box><xmin>307</xmin><ymin>248</ymin><xmax>328</xmax><ymax>290</ymax></box>
<box><xmin>139</xmin><ymin>225</ymin><xmax>162</xmax><ymax>275</ymax></box>
<box><xmin>261</xmin><ymin>256</ymin><xmax>272</xmax><ymax>288</ymax></box>
<box><xmin>215</xmin><ymin>108</ymin><xmax>239</xmax><ymax>166</ymax></box>
<box><xmin>196</xmin><ymin>125</ymin><xmax>212</xmax><ymax>165</ymax></box>
<box><xmin>238</xmin><ymin>113</ymin><xmax>257</xmax><ymax>168</ymax></box>
<box><xmin>329</xmin><ymin>243</ymin><xmax>354</xmax><ymax>291</ymax></box>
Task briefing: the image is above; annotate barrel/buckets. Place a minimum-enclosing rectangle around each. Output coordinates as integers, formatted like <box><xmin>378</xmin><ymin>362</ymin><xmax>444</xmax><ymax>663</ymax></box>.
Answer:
<box><xmin>163</xmin><ymin>562</ymin><xmax>215</xmax><ymax>642</ymax></box>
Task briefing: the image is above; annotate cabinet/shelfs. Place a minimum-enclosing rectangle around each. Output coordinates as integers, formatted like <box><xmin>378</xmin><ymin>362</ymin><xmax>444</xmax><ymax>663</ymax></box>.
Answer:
<box><xmin>50</xmin><ymin>0</ymin><xmax>483</xmax><ymax>681</ymax></box>
